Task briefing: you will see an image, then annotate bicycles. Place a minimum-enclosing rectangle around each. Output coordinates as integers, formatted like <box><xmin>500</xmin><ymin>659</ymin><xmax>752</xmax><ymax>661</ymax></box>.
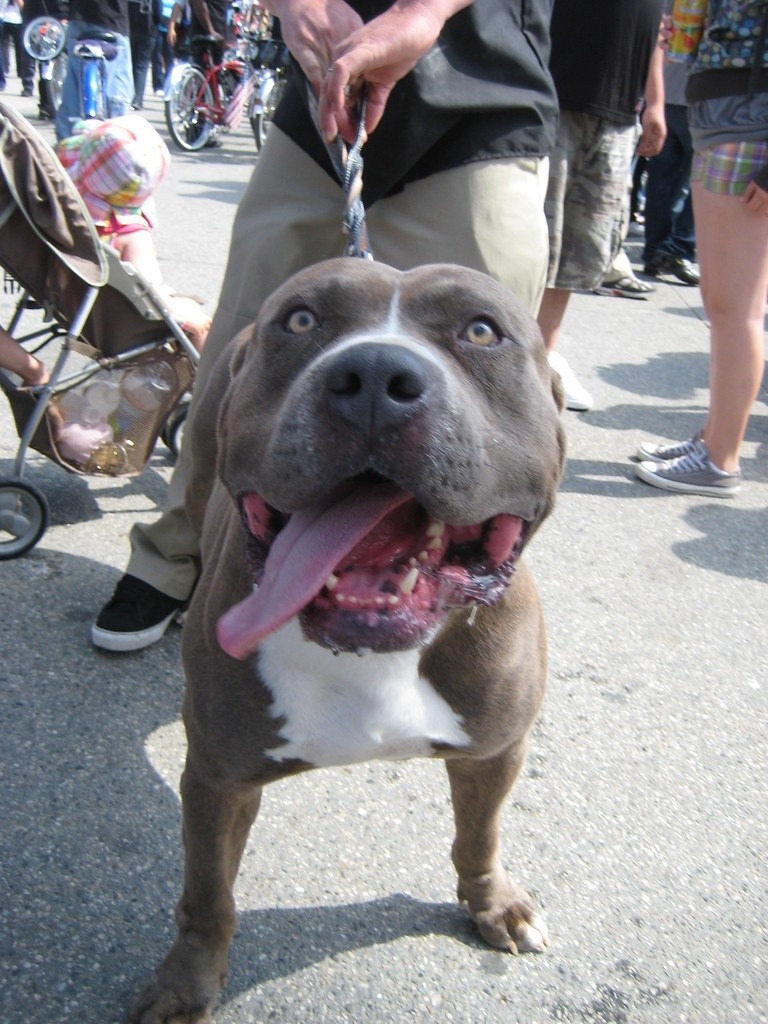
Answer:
<box><xmin>76</xmin><ymin>26</ymin><xmax>119</xmax><ymax>122</ymax></box>
<box><xmin>160</xmin><ymin>11</ymin><xmax>280</xmax><ymax>153</ymax></box>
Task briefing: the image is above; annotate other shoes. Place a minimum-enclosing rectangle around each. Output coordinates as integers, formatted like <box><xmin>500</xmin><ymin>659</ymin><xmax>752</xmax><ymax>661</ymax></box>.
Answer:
<box><xmin>645</xmin><ymin>253</ymin><xmax>700</xmax><ymax>285</ymax></box>
<box><xmin>545</xmin><ymin>350</ymin><xmax>589</xmax><ymax>410</ymax></box>
<box><xmin>132</xmin><ymin>99</ymin><xmax>144</xmax><ymax>111</ymax></box>
<box><xmin>154</xmin><ymin>89</ymin><xmax>164</xmax><ymax>98</ymax></box>
<box><xmin>38</xmin><ymin>109</ymin><xmax>55</xmax><ymax>120</ymax></box>
<box><xmin>628</xmin><ymin>220</ymin><xmax>645</xmax><ymax>237</ymax></box>
<box><xmin>187</xmin><ymin>132</ymin><xmax>222</xmax><ymax>148</ymax></box>
<box><xmin>22</xmin><ymin>90</ymin><xmax>33</xmax><ymax>97</ymax></box>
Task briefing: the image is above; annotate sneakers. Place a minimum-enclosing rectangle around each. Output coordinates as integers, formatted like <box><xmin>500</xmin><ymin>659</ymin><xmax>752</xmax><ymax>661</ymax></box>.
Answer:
<box><xmin>637</xmin><ymin>428</ymin><xmax>707</xmax><ymax>461</ymax></box>
<box><xmin>92</xmin><ymin>571</ymin><xmax>181</xmax><ymax>651</ymax></box>
<box><xmin>594</xmin><ymin>275</ymin><xmax>655</xmax><ymax>298</ymax></box>
<box><xmin>634</xmin><ymin>444</ymin><xmax>741</xmax><ymax>498</ymax></box>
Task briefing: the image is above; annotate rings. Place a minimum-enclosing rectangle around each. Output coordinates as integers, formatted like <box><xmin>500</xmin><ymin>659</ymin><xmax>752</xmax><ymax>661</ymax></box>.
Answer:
<box><xmin>326</xmin><ymin>68</ymin><xmax>332</xmax><ymax>72</ymax></box>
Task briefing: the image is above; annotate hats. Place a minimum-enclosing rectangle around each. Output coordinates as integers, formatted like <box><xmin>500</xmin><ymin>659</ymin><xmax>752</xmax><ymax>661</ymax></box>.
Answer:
<box><xmin>57</xmin><ymin>118</ymin><xmax>173</xmax><ymax>234</ymax></box>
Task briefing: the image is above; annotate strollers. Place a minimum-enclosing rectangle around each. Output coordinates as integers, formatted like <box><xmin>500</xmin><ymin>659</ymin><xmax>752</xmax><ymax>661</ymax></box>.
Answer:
<box><xmin>0</xmin><ymin>101</ymin><xmax>223</xmax><ymax>561</ymax></box>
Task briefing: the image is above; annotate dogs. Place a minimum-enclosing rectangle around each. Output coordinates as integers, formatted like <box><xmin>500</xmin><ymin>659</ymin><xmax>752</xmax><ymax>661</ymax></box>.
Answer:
<box><xmin>119</xmin><ymin>253</ymin><xmax>568</xmax><ymax>1024</ymax></box>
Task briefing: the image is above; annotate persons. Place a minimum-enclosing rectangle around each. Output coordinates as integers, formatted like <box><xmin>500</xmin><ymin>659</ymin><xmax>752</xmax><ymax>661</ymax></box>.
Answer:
<box><xmin>90</xmin><ymin>0</ymin><xmax>559</xmax><ymax>652</ymax></box>
<box><xmin>0</xmin><ymin>0</ymin><xmax>283</xmax><ymax>445</ymax></box>
<box><xmin>538</xmin><ymin>1</ymin><xmax>768</xmax><ymax>499</ymax></box>
